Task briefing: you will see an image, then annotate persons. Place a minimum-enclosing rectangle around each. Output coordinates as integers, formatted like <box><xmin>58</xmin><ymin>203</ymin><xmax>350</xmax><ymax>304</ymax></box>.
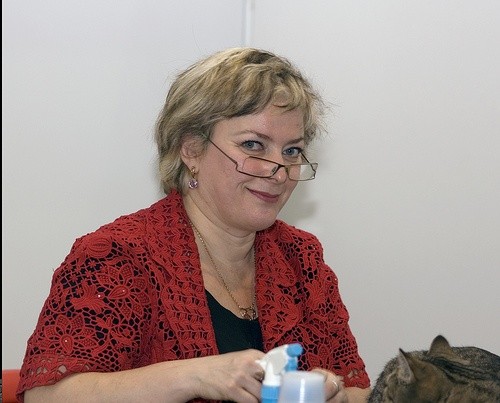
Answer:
<box><xmin>16</xmin><ymin>47</ymin><xmax>371</xmax><ymax>403</ymax></box>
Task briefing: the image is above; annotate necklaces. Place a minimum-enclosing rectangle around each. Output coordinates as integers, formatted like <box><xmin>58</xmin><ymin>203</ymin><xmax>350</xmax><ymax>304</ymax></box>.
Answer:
<box><xmin>189</xmin><ymin>220</ymin><xmax>257</xmax><ymax>320</ymax></box>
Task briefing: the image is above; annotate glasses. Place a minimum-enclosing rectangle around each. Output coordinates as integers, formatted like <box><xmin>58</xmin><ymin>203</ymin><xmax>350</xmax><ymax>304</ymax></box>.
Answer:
<box><xmin>201</xmin><ymin>132</ymin><xmax>318</xmax><ymax>181</ymax></box>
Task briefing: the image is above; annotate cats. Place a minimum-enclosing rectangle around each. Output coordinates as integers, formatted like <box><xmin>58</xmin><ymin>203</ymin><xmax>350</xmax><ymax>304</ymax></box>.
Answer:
<box><xmin>368</xmin><ymin>335</ymin><xmax>500</xmax><ymax>403</ymax></box>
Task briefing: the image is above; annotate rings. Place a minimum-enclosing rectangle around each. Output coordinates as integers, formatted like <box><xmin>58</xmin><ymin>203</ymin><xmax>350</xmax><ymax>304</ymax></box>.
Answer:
<box><xmin>332</xmin><ymin>380</ymin><xmax>340</xmax><ymax>393</ymax></box>
<box><xmin>254</xmin><ymin>366</ymin><xmax>265</xmax><ymax>382</ymax></box>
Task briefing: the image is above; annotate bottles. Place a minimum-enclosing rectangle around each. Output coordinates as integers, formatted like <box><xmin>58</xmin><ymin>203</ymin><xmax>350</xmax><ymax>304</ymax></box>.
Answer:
<box><xmin>278</xmin><ymin>371</ymin><xmax>327</xmax><ymax>403</ymax></box>
